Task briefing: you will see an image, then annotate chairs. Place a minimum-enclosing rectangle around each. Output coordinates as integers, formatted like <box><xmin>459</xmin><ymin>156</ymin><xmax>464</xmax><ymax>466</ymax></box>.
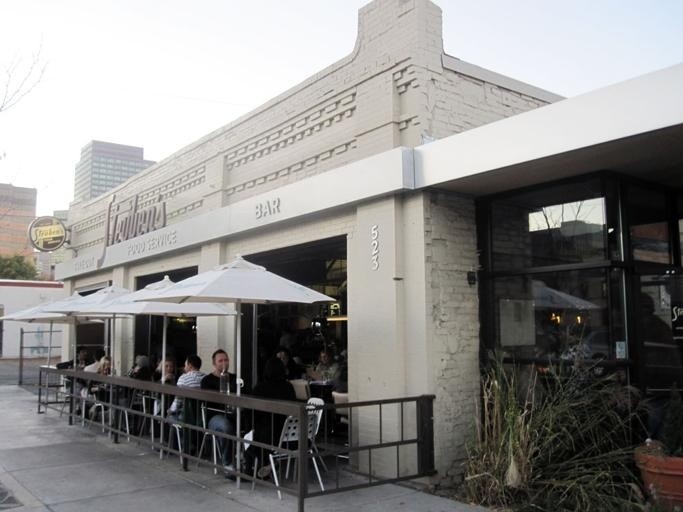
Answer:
<box><xmin>57</xmin><ymin>376</ymin><xmax>349</xmax><ymax>501</ymax></box>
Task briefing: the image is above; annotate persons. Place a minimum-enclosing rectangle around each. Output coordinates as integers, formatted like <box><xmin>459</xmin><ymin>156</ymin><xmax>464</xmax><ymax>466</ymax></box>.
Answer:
<box><xmin>304</xmin><ymin>349</ymin><xmax>343</xmax><ymax>394</ymax></box>
<box><xmin>271</xmin><ymin>345</ymin><xmax>304</xmax><ymax>379</ymax></box>
<box><xmin>52</xmin><ymin>342</ymin><xmax>206</xmax><ymax>456</ymax></box>
<box><xmin>640</xmin><ymin>286</ymin><xmax>673</xmax><ymax>378</ymax></box>
<box><xmin>199</xmin><ymin>347</ymin><xmax>251</xmax><ymax>479</ymax></box>
<box><xmin>242</xmin><ymin>355</ymin><xmax>296</xmax><ymax>482</ymax></box>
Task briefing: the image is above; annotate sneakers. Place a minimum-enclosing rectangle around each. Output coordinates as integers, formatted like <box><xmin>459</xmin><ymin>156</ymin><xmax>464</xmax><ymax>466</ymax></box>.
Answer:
<box><xmin>258</xmin><ymin>464</ymin><xmax>271</xmax><ymax>478</ymax></box>
<box><xmin>225</xmin><ymin>464</ymin><xmax>236</xmax><ymax>473</ymax></box>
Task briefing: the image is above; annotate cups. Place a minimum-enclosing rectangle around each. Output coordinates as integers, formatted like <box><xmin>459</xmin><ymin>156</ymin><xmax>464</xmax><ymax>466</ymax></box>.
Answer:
<box><xmin>301</xmin><ymin>373</ymin><xmax>308</xmax><ymax>383</ymax></box>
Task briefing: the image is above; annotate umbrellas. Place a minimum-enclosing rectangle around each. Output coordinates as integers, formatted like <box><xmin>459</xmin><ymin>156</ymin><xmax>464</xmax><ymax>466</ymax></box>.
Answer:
<box><xmin>85</xmin><ymin>271</ymin><xmax>240</xmax><ymax>463</ymax></box>
<box><xmin>44</xmin><ymin>283</ymin><xmax>145</xmax><ymax>439</ymax></box>
<box><xmin>0</xmin><ymin>292</ymin><xmax>101</xmax><ymax>401</ymax></box>
<box><xmin>128</xmin><ymin>251</ymin><xmax>336</xmax><ymax>485</ymax></box>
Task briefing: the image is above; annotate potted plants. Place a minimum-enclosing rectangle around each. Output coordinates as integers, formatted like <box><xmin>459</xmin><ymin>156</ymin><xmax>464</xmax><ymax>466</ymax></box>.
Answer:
<box><xmin>632</xmin><ymin>381</ymin><xmax>683</xmax><ymax>512</ymax></box>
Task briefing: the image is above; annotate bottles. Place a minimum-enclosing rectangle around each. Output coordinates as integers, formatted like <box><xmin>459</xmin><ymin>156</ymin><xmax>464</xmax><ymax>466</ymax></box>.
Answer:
<box><xmin>102</xmin><ymin>362</ymin><xmax>107</xmax><ymax>375</ymax></box>
<box><xmin>78</xmin><ymin>360</ymin><xmax>86</xmax><ymax>370</ymax></box>
<box><xmin>320</xmin><ymin>366</ymin><xmax>334</xmax><ymax>385</ymax></box>
<box><xmin>219</xmin><ymin>366</ymin><xmax>231</xmax><ymax>394</ymax></box>
